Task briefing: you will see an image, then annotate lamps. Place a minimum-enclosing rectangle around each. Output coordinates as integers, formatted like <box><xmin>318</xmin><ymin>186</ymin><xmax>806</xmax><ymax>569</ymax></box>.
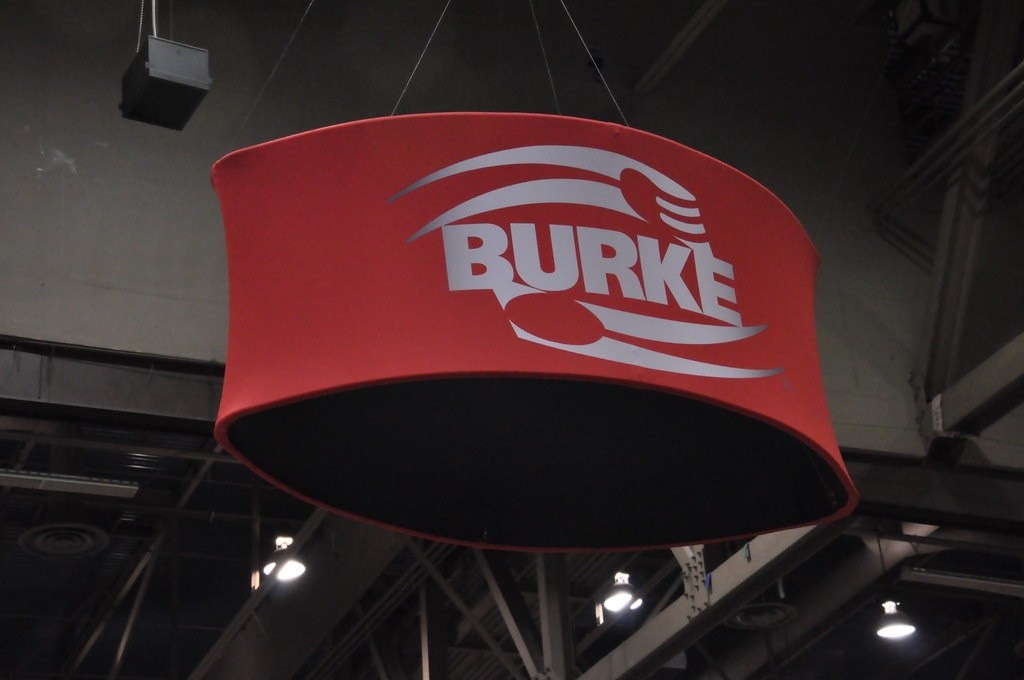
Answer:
<box><xmin>876</xmin><ymin>599</ymin><xmax>919</xmax><ymax>642</ymax></box>
<box><xmin>264</xmin><ymin>536</ymin><xmax>307</xmax><ymax>584</ymax></box>
<box><xmin>595</xmin><ymin>570</ymin><xmax>643</xmax><ymax>615</ymax></box>
